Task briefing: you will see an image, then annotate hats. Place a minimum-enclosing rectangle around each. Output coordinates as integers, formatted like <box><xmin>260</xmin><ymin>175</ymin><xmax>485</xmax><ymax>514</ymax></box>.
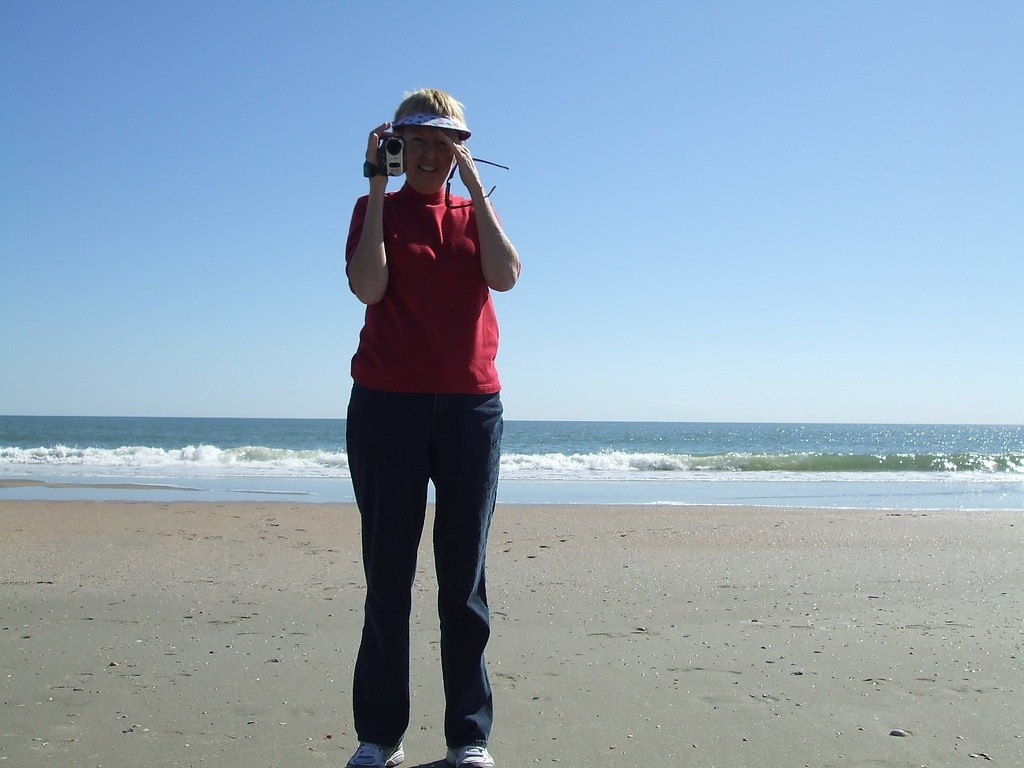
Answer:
<box><xmin>391</xmin><ymin>112</ymin><xmax>472</xmax><ymax>141</ymax></box>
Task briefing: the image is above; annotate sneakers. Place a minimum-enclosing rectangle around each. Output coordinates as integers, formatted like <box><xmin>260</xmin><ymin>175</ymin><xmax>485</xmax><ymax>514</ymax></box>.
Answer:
<box><xmin>346</xmin><ymin>737</ymin><xmax>404</xmax><ymax>768</ymax></box>
<box><xmin>446</xmin><ymin>745</ymin><xmax>495</xmax><ymax>768</ymax></box>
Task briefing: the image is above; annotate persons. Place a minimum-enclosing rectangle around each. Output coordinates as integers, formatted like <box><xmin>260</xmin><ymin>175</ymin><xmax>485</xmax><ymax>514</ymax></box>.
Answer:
<box><xmin>344</xmin><ymin>90</ymin><xmax>522</xmax><ymax>768</ymax></box>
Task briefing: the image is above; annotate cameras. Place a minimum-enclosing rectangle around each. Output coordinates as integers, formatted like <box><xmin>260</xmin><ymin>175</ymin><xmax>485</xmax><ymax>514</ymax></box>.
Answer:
<box><xmin>384</xmin><ymin>137</ymin><xmax>407</xmax><ymax>176</ymax></box>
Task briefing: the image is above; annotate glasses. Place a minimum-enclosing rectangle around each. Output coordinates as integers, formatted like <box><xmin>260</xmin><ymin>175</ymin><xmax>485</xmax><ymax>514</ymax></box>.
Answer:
<box><xmin>445</xmin><ymin>157</ymin><xmax>510</xmax><ymax>209</ymax></box>
